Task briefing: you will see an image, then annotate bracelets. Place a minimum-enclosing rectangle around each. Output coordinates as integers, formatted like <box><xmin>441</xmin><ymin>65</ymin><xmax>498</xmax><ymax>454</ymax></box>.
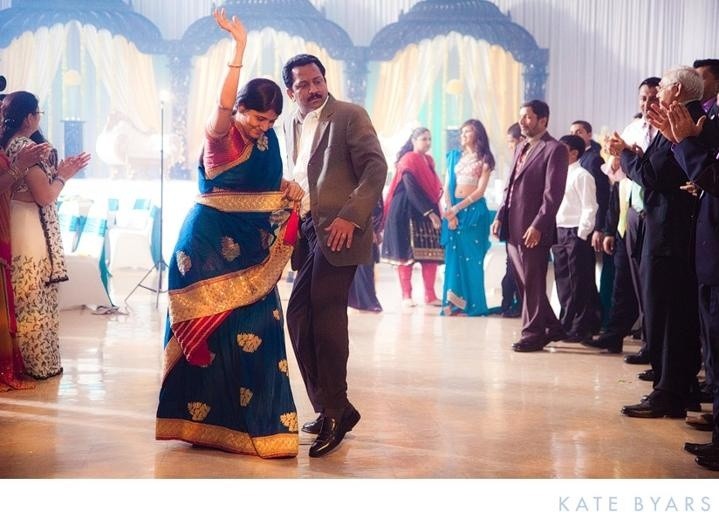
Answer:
<box><xmin>228</xmin><ymin>62</ymin><xmax>243</xmax><ymax>69</ymax></box>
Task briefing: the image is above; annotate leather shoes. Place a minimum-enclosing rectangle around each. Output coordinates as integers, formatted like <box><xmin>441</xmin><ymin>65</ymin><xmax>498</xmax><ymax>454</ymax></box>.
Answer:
<box><xmin>622</xmin><ymin>354</ymin><xmax>719</xmax><ymax>471</ymax></box>
<box><xmin>301</xmin><ymin>403</ymin><xmax>360</xmax><ymax>457</ymax></box>
<box><xmin>512</xmin><ymin>323</ymin><xmax>622</xmax><ymax>354</ymax></box>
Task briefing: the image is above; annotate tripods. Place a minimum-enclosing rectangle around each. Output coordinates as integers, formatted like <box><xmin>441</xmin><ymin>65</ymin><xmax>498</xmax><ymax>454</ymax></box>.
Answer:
<box><xmin>124</xmin><ymin>102</ymin><xmax>169</xmax><ymax>308</ymax></box>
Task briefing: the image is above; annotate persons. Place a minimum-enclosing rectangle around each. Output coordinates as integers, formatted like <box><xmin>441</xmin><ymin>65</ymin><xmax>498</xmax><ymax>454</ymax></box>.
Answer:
<box><xmin>437</xmin><ymin>118</ymin><xmax>492</xmax><ymax>318</ymax></box>
<box><xmin>349</xmin><ymin>194</ymin><xmax>384</xmax><ymax>312</ymax></box>
<box><xmin>501</xmin><ymin>121</ymin><xmax>527</xmax><ymax>318</ymax></box>
<box><xmin>0</xmin><ymin>74</ymin><xmax>91</xmax><ymax>393</ymax></box>
<box><xmin>156</xmin><ymin>7</ymin><xmax>305</xmax><ymax>459</ymax></box>
<box><xmin>383</xmin><ymin>127</ymin><xmax>443</xmax><ymax>307</ymax></box>
<box><xmin>282</xmin><ymin>55</ymin><xmax>387</xmax><ymax>458</ymax></box>
<box><xmin>554</xmin><ymin>59</ymin><xmax>718</xmax><ymax>472</ymax></box>
<box><xmin>491</xmin><ymin>99</ymin><xmax>569</xmax><ymax>350</ymax></box>
